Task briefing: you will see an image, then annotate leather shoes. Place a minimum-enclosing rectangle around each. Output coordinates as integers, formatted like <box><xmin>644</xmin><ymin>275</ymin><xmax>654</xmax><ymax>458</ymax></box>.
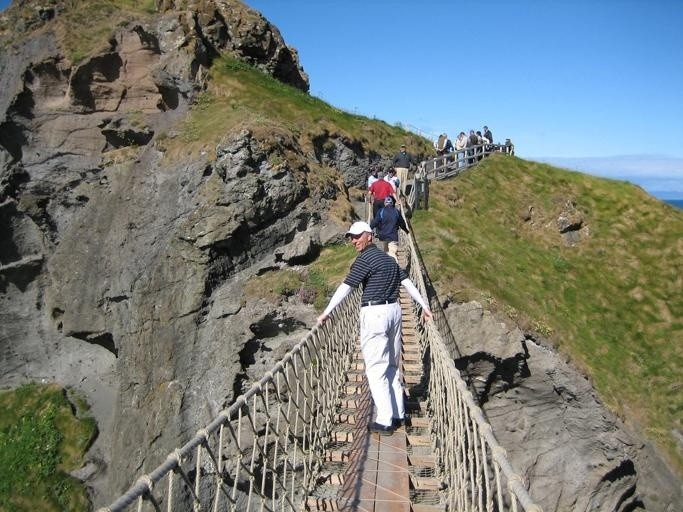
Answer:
<box><xmin>393</xmin><ymin>418</ymin><xmax>405</xmax><ymax>426</ymax></box>
<box><xmin>368</xmin><ymin>422</ymin><xmax>392</xmax><ymax>436</ymax></box>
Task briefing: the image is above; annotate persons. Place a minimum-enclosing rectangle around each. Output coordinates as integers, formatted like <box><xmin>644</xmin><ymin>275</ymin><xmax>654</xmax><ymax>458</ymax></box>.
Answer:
<box><xmin>312</xmin><ymin>218</ymin><xmax>436</xmax><ymax>439</ymax></box>
<box><xmin>360</xmin><ymin>125</ymin><xmax>492</xmax><ymax>239</ymax></box>
<box><xmin>369</xmin><ymin>195</ymin><xmax>409</xmax><ymax>265</ymax></box>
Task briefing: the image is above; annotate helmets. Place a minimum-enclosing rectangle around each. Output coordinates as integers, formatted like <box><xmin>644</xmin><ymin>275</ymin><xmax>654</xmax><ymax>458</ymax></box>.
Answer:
<box><xmin>384</xmin><ymin>196</ymin><xmax>393</xmax><ymax>203</ymax></box>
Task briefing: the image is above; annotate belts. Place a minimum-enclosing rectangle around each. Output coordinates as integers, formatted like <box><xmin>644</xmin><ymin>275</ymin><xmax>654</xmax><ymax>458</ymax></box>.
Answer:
<box><xmin>361</xmin><ymin>299</ymin><xmax>396</xmax><ymax>307</ymax></box>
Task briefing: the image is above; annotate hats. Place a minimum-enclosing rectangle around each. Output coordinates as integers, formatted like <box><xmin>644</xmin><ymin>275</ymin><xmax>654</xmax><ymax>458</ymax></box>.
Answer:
<box><xmin>344</xmin><ymin>221</ymin><xmax>372</xmax><ymax>238</ymax></box>
<box><xmin>400</xmin><ymin>144</ymin><xmax>405</xmax><ymax>147</ymax></box>
<box><xmin>369</xmin><ymin>167</ymin><xmax>394</xmax><ymax>177</ymax></box>
